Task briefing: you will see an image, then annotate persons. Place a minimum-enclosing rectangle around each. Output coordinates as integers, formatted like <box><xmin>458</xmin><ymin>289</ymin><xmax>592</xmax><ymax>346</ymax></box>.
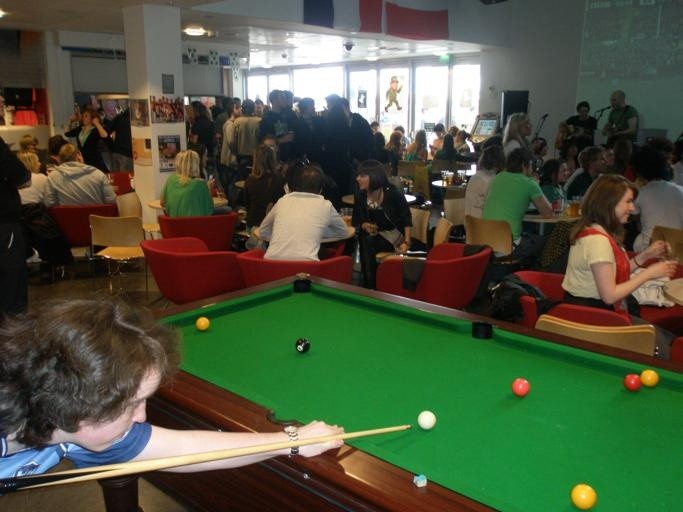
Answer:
<box><xmin>0</xmin><ymin>89</ymin><xmax>682</xmax><ymax>361</ymax></box>
<box><xmin>383</xmin><ymin>76</ymin><xmax>404</xmax><ymax>112</ymax></box>
<box><xmin>1</xmin><ymin>290</ymin><xmax>346</xmax><ymax>492</ymax></box>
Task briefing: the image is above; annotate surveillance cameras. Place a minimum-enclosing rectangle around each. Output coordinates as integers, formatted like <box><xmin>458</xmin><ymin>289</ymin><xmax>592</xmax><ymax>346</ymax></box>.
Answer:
<box><xmin>343</xmin><ymin>44</ymin><xmax>354</xmax><ymax>51</ymax></box>
<box><xmin>281</xmin><ymin>54</ymin><xmax>288</xmax><ymax>59</ymax></box>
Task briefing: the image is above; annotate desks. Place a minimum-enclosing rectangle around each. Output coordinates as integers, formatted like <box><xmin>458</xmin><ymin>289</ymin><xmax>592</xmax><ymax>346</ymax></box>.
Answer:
<box><xmin>89</xmin><ymin>272</ymin><xmax>683</xmax><ymax>511</ymax></box>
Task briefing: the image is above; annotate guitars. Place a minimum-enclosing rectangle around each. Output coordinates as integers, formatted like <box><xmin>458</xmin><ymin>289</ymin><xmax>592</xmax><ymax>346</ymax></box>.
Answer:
<box><xmin>554</xmin><ymin>125</ymin><xmax>595</xmax><ymax>149</ymax></box>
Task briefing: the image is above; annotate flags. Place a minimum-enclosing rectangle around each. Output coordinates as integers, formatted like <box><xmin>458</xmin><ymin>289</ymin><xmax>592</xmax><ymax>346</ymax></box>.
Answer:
<box><xmin>304</xmin><ymin>0</ymin><xmax>384</xmax><ymax>34</ymax></box>
<box><xmin>387</xmin><ymin>0</ymin><xmax>448</xmax><ymax>40</ymax></box>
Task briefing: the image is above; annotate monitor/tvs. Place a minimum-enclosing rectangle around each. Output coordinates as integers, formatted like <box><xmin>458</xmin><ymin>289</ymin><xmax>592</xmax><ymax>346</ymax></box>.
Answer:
<box><xmin>470</xmin><ymin>117</ymin><xmax>500</xmax><ymax>138</ymax></box>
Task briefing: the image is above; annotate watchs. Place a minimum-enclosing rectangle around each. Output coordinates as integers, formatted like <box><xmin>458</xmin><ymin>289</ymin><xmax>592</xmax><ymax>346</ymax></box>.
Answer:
<box><xmin>282</xmin><ymin>425</ymin><xmax>301</xmax><ymax>459</ymax></box>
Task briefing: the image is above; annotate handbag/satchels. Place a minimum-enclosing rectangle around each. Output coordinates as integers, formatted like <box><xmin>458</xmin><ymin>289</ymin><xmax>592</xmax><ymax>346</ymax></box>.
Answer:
<box><xmin>188</xmin><ymin>135</ymin><xmax>204</xmax><ymax>154</ymax></box>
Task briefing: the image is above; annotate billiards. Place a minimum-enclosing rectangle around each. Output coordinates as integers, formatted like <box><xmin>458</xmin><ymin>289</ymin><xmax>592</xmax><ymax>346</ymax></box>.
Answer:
<box><xmin>196</xmin><ymin>317</ymin><xmax>210</xmax><ymax>330</ymax></box>
<box><xmin>571</xmin><ymin>484</ymin><xmax>596</xmax><ymax>509</ymax></box>
<box><xmin>295</xmin><ymin>339</ymin><xmax>310</xmax><ymax>352</ymax></box>
<box><xmin>418</xmin><ymin>410</ymin><xmax>436</xmax><ymax>430</ymax></box>
<box><xmin>640</xmin><ymin>370</ymin><xmax>659</xmax><ymax>387</ymax></box>
<box><xmin>512</xmin><ymin>377</ymin><xmax>530</xmax><ymax>396</ymax></box>
<box><xmin>624</xmin><ymin>374</ymin><xmax>641</xmax><ymax>391</ymax></box>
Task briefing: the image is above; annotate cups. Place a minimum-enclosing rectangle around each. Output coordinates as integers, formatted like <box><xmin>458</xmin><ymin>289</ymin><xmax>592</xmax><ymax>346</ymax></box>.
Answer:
<box><xmin>568</xmin><ymin>200</ymin><xmax>580</xmax><ymax>217</ymax></box>
<box><xmin>649</xmin><ymin>232</ymin><xmax>668</xmax><ymax>262</ymax></box>
<box><xmin>440</xmin><ymin>170</ymin><xmax>449</xmax><ymax>182</ymax></box>
<box><xmin>446</xmin><ymin>173</ymin><xmax>454</xmax><ymax>185</ymax></box>
<box><xmin>369</xmin><ymin>222</ymin><xmax>378</xmax><ymax>236</ymax></box>
<box><xmin>217</xmin><ymin>188</ymin><xmax>224</xmax><ymax>201</ymax></box>
<box><xmin>340</xmin><ymin>208</ymin><xmax>354</xmax><ymax>226</ymax></box>
<box><xmin>458</xmin><ymin>173</ymin><xmax>464</xmax><ymax>182</ymax></box>
<box><xmin>554</xmin><ymin>199</ymin><xmax>564</xmax><ymax>216</ymax></box>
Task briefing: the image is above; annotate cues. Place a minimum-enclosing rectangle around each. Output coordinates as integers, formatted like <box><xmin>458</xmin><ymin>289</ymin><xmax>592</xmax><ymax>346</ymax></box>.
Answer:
<box><xmin>1</xmin><ymin>424</ymin><xmax>411</xmax><ymax>494</ymax></box>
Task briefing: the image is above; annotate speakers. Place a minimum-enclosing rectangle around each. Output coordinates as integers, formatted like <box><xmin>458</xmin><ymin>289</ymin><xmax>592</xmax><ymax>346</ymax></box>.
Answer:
<box><xmin>501</xmin><ymin>89</ymin><xmax>529</xmax><ymax>130</ymax></box>
